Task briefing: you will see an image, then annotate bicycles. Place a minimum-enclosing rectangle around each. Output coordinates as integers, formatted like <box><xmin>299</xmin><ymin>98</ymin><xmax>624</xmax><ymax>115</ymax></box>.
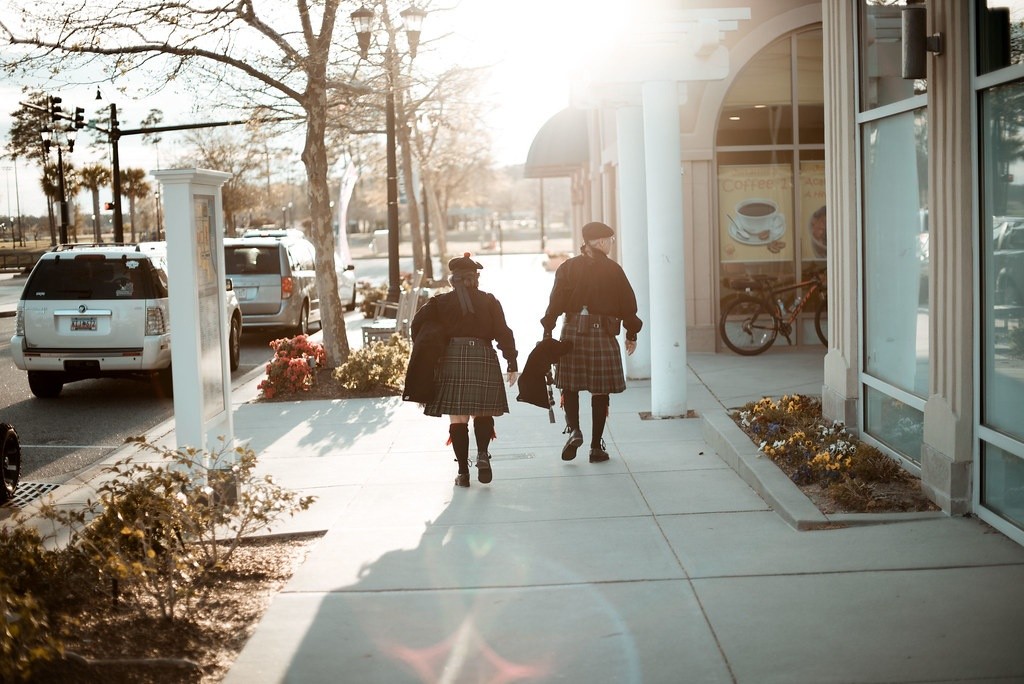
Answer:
<box><xmin>718</xmin><ymin>267</ymin><xmax>830</xmax><ymax>356</ymax></box>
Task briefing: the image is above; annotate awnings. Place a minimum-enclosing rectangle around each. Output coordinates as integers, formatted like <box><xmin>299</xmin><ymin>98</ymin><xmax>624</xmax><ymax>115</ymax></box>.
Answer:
<box><xmin>522</xmin><ymin>104</ymin><xmax>587</xmax><ymax>179</ymax></box>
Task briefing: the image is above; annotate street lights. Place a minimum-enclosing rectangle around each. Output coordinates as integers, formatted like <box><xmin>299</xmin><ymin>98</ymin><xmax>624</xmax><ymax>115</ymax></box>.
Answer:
<box><xmin>10</xmin><ymin>218</ymin><xmax>15</xmax><ymax>249</ymax></box>
<box><xmin>154</xmin><ymin>191</ymin><xmax>160</xmax><ymax>241</ymax></box>
<box><xmin>36</xmin><ymin>123</ymin><xmax>79</xmax><ymax>242</ymax></box>
<box><xmin>19</xmin><ymin>100</ymin><xmax>125</xmax><ymax>247</ymax></box>
<box><xmin>91</xmin><ymin>215</ymin><xmax>95</xmax><ymax>242</ymax></box>
<box><xmin>349</xmin><ymin>2</ymin><xmax>429</xmax><ymax>318</ymax></box>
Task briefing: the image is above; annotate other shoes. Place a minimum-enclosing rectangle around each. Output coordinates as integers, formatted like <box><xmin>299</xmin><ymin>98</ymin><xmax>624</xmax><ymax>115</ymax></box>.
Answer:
<box><xmin>477</xmin><ymin>452</ymin><xmax>492</xmax><ymax>483</ymax></box>
<box><xmin>454</xmin><ymin>473</ymin><xmax>470</xmax><ymax>487</ymax></box>
<box><xmin>560</xmin><ymin>430</ymin><xmax>583</xmax><ymax>461</ymax></box>
<box><xmin>589</xmin><ymin>447</ymin><xmax>609</xmax><ymax>463</ymax></box>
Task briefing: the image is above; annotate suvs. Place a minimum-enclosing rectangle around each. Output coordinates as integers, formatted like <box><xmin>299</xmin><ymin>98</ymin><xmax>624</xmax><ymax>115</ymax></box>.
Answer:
<box><xmin>10</xmin><ymin>243</ymin><xmax>242</xmax><ymax>401</ymax></box>
<box><xmin>994</xmin><ymin>216</ymin><xmax>1024</xmax><ymax>317</ymax></box>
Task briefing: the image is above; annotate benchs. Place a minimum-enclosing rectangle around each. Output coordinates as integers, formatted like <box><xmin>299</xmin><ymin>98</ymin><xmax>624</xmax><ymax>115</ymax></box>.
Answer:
<box><xmin>362</xmin><ymin>267</ymin><xmax>424</xmax><ymax>347</ymax></box>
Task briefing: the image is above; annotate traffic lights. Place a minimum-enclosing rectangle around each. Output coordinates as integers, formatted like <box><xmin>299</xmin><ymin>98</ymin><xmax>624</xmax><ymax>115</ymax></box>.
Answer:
<box><xmin>47</xmin><ymin>94</ymin><xmax>62</xmax><ymax>121</ymax></box>
<box><xmin>104</xmin><ymin>202</ymin><xmax>113</xmax><ymax>211</ymax></box>
<box><xmin>74</xmin><ymin>105</ymin><xmax>85</xmax><ymax>129</ymax></box>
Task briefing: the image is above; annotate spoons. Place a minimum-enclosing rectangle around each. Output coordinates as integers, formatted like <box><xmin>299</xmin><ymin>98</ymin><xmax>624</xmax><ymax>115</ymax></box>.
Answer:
<box><xmin>728</xmin><ymin>216</ymin><xmax>750</xmax><ymax>239</ymax></box>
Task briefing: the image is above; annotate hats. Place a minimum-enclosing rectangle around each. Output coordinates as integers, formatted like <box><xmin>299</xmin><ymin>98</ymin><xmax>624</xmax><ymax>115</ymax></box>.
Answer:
<box><xmin>582</xmin><ymin>222</ymin><xmax>614</xmax><ymax>241</ymax></box>
<box><xmin>449</xmin><ymin>252</ymin><xmax>483</xmax><ymax>271</ymax></box>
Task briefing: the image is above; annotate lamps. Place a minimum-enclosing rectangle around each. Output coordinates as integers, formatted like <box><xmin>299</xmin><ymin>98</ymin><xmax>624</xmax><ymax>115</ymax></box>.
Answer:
<box><xmin>900</xmin><ymin>8</ymin><xmax>945</xmax><ymax>79</ymax></box>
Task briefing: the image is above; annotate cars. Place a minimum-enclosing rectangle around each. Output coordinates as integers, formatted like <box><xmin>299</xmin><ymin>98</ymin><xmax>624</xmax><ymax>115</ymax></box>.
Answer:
<box><xmin>223</xmin><ymin>230</ymin><xmax>357</xmax><ymax>339</ymax></box>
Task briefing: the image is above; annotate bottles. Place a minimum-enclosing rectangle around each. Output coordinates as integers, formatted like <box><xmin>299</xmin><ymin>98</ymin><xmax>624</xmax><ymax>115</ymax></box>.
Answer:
<box><xmin>788</xmin><ymin>296</ymin><xmax>802</xmax><ymax>314</ymax></box>
<box><xmin>580</xmin><ymin>305</ymin><xmax>590</xmax><ymax>315</ymax></box>
<box><xmin>776</xmin><ymin>297</ymin><xmax>785</xmax><ymax>317</ymax></box>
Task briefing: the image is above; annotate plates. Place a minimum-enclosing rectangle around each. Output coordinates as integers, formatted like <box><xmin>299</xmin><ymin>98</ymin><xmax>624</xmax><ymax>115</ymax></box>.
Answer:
<box><xmin>728</xmin><ymin>212</ymin><xmax>786</xmax><ymax>245</ymax></box>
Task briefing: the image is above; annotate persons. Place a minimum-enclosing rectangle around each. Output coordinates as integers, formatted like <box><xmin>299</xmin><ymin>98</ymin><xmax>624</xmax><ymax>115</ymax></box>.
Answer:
<box><xmin>540</xmin><ymin>222</ymin><xmax>643</xmax><ymax>464</ymax></box>
<box><xmin>411</xmin><ymin>252</ymin><xmax>519</xmax><ymax>486</ymax></box>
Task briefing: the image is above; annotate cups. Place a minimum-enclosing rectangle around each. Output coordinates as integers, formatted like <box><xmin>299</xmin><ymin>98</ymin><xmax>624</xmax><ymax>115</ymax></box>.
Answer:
<box><xmin>735</xmin><ymin>198</ymin><xmax>785</xmax><ymax>235</ymax></box>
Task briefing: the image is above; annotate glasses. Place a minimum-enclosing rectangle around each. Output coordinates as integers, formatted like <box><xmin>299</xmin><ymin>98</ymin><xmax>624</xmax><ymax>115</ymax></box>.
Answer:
<box><xmin>604</xmin><ymin>236</ymin><xmax>616</xmax><ymax>244</ymax></box>
